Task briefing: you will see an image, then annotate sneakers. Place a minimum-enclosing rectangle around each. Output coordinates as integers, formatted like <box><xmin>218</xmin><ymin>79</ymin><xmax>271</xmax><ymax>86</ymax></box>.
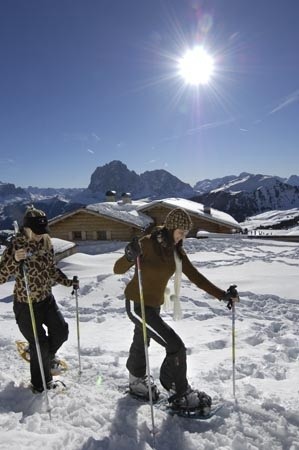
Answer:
<box><xmin>32</xmin><ymin>381</ymin><xmax>58</xmax><ymax>394</ymax></box>
<box><xmin>48</xmin><ymin>358</ymin><xmax>60</xmax><ymax>369</ymax></box>
<box><xmin>168</xmin><ymin>388</ymin><xmax>212</xmax><ymax>412</ymax></box>
<box><xmin>129</xmin><ymin>372</ymin><xmax>160</xmax><ymax>400</ymax></box>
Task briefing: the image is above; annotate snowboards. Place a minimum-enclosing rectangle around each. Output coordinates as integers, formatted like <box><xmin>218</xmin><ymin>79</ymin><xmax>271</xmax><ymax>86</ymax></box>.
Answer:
<box><xmin>16</xmin><ymin>340</ymin><xmax>69</xmax><ymax>397</ymax></box>
<box><xmin>122</xmin><ymin>378</ymin><xmax>224</xmax><ymax>420</ymax></box>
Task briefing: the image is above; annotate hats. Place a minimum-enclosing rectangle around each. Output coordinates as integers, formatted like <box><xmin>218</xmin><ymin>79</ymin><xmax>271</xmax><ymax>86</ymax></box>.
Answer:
<box><xmin>164</xmin><ymin>207</ymin><xmax>192</xmax><ymax>230</ymax></box>
<box><xmin>23</xmin><ymin>216</ymin><xmax>51</xmax><ymax>235</ymax></box>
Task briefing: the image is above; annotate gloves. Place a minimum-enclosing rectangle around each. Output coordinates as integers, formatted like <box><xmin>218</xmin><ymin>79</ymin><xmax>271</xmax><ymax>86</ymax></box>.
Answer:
<box><xmin>125</xmin><ymin>242</ymin><xmax>144</xmax><ymax>262</ymax></box>
<box><xmin>223</xmin><ymin>289</ymin><xmax>240</xmax><ymax>302</ymax></box>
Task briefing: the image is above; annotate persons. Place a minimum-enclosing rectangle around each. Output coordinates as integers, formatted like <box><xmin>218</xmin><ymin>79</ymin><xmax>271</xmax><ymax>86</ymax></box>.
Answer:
<box><xmin>113</xmin><ymin>207</ymin><xmax>238</xmax><ymax>411</ymax></box>
<box><xmin>0</xmin><ymin>210</ymin><xmax>80</xmax><ymax>394</ymax></box>
